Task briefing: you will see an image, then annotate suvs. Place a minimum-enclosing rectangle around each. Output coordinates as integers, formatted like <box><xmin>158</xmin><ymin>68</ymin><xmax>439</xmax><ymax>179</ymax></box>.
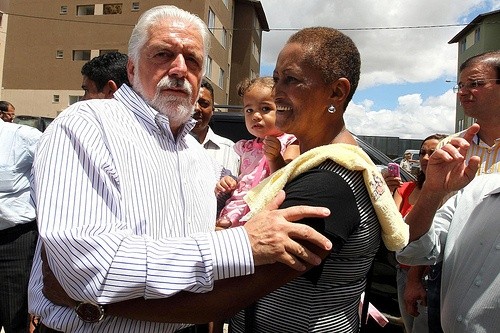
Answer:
<box><xmin>207</xmin><ymin>104</ymin><xmax>418</xmax><ymax>328</ymax></box>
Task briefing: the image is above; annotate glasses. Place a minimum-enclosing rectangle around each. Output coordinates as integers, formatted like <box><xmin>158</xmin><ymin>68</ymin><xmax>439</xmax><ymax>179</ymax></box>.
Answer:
<box><xmin>453</xmin><ymin>78</ymin><xmax>500</xmax><ymax>94</ymax></box>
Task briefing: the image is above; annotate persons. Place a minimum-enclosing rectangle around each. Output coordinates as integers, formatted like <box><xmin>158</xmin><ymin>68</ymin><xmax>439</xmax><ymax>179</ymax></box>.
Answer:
<box><xmin>395</xmin><ymin>123</ymin><xmax>500</xmax><ymax>333</ymax></box>
<box><xmin>82</xmin><ymin>52</ymin><xmax>129</xmax><ymax>101</ymax></box>
<box><xmin>215</xmin><ymin>75</ymin><xmax>300</xmax><ymax>231</ymax></box>
<box><xmin>0</xmin><ymin>100</ymin><xmax>16</xmax><ymax>123</ymax></box>
<box><xmin>434</xmin><ymin>51</ymin><xmax>500</xmax><ymax>204</ymax></box>
<box><xmin>400</xmin><ymin>153</ymin><xmax>412</xmax><ymax>173</ymax></box>
<box><xmin>41</xmin><ymin>26</ymin><xmax>382</xmax><ymax>333</ymax></box>
<box><xmin>27</xmin><ymin>5</ymin><xmax>332</xmax><ymax>333</ymax></box>
<box><xmin>189</xmin><ymin>79</ymin><xmax>241</xmax><ymax>182</ymax></box>
<box><xmin>0</xmin><ymin>123</ymin><xmax>44</xmax><ymax>333</ymax></box>
<box><xmin>381</xmin><ymin>134</ymin><xmax>446</xmax><ymax>333</ymax></box>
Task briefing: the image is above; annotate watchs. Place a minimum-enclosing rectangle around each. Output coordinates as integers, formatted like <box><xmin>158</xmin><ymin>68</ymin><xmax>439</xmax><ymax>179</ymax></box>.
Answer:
<box><xmin>76</xmin><ymin>302</ymin><xmax>104</xmax><ymax>323</ymax></box>
